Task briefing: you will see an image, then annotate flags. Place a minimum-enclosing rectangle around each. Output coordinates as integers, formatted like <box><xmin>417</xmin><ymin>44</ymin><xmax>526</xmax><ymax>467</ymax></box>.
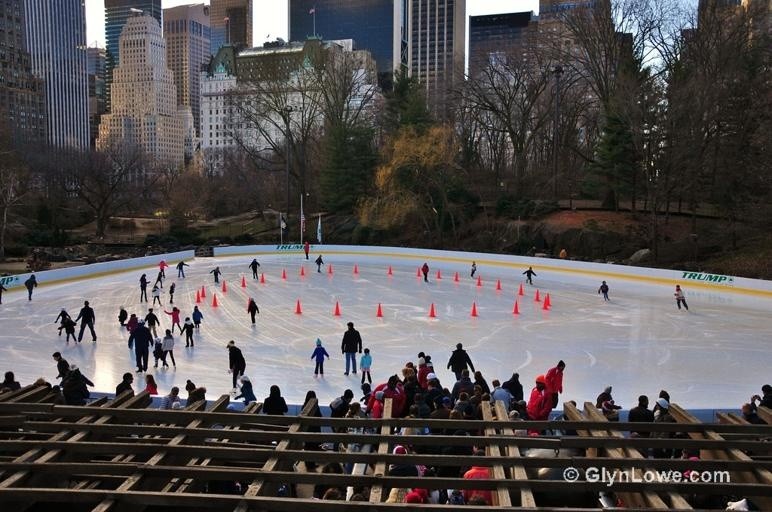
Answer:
<box><xmin>223</xmin><ymin>16</ymin><xmax>230</xmax><ymax>22</ymax></box>
<box><xmin>301</xmin><ymin>214</ymin><xmax>306</xmax><ymax>233</ymax></box>
<box><xmin>308</xmin><ymin>8</ymin><xmax>315</xmax><ymax>15</ymax></box>
<box><xmin>281</xmin><ymin>216</ymin><xmax>287</xmax><ymax>229</ymax></box>
<box><xmin>317</xmin><ymin>217</ymin><xmax>321</xmax><ymax>243</ymax></box>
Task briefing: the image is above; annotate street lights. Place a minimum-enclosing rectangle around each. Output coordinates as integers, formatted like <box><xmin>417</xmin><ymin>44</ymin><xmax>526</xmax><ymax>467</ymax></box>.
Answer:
<box><xmin>547</xmin><ymin>60</ymin><xmax>563</xmax><ymax>207</ymax></box>
<box><xmin>281</xmin><ymin>103</ymin><xmax>293</xmax><ymax>242</ymax></box>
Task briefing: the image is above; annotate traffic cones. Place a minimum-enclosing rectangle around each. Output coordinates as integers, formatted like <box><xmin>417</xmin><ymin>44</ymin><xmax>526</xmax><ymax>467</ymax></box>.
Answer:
<box><xmin>386</xmin><ymin>265</ymin><xmax>392</xmax><ymax>274</ymax></box>
<box><xmin>327</xmin><ymin>263</ymin><xmax>334</xmax><ymax>275</ymax></box>
<box><xmin>417</xmin><ymin>263</ymin><xmax>555</xmax><ymax>318</ymax></box>
<box><xmin>196</xmin><ymin>267</ymin><xmax>247</xmax><ymax>308</ymax></box>
<box><xmin>259</xmin><ymin>272</ymin><xmax>265</xmax><ymax>284</ymax></box>
<box><xmin>295</xmin><ymin>298</ymin><xmax>304</xmax><ymax>314</ymax></box>
<box><xmin>299</xmin><ymin>265</ymin><xmax>306</xmax><ymax>276</ymax></box>
<box><xmin>375</xmin><ymin>302</ymin><xmax>384</xmax><ymax>317</ymax></box>
<box><xmin>281</xmin><ymin>269</ymin><xmax>287</xmax><ymax>279</ymax></box>
<box><xmin>333</xmin><ymin>300</ymin><xmax>342</xmax><ymax>316</ymax></box>
<box><xmin>353</xmin><ymin>262</ymin><xmax>360</xmax><ymax>274</ymax></box>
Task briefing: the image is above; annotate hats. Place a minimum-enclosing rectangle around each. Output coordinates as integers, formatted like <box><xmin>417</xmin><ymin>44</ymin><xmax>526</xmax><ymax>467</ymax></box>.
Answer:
<box><xmin>426</xmin><ymin>373</ymin><xmax>435</xmax><ymax>379</ymax></box>
<box><xmin>657</xmin><ymin>398</ymin><xmax>668</xmax><ymax>408</ymax></box>
